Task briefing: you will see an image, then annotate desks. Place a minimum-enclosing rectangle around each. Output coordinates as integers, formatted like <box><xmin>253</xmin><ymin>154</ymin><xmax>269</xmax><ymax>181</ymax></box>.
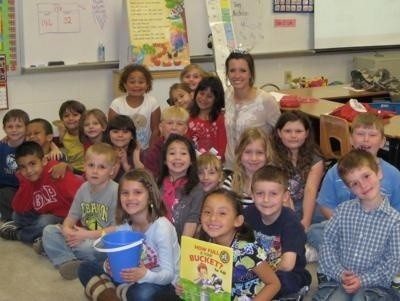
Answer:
<box><xmin>276</xmin><ymin>97</ymin><xmax>400</xmax><ymax>166</ymax></box>
<box><xmin>263</xmin><ymin>83</ymin><xmax>392</xmax><ymax>102</ymax></box>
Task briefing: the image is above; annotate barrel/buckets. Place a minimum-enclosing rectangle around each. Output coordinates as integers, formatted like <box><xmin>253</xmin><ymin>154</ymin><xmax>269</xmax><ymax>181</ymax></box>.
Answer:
<box><xmin>92</xmin><ymin>230</ymin><xmax>146</xmax><ymax>285</ymax></box>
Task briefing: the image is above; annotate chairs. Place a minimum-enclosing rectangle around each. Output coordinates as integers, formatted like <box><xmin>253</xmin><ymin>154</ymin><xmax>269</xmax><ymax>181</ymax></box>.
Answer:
<box><xmin>259</xmin><ymin>82</ymin><xmax>280</xmax><ymax>92</ymax></box>
<box><xmin>317</xmin><ymin>113</ymin><xmax>353</xmax><ymax>162</ymax></box>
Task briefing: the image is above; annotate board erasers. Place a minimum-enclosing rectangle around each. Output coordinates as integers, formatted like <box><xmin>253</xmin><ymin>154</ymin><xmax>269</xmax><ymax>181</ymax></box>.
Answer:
<box><xmin>48</xmin><ymin>61</ymin><xmax>65</xmax><ymax>66</ymax></box>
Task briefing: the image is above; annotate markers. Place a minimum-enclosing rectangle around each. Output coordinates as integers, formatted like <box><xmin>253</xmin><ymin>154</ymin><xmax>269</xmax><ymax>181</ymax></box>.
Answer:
<box><xmin>29</xmin><ymin>64</ymin><xmax>46</xmax><ymax>68</ymax></box>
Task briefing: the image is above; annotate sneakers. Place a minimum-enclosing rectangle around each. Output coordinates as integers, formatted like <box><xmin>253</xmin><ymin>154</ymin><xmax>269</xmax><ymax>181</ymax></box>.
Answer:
<box><xmin>59</xmin><ymin>259</ymin><xmax>89</xmax><ymax>281</ymax></box>
<box><xmin>0</xmin><ymin>220</ymin><xmax>26</xmax><ymax>241</ymax></box>
<box><xmin>33</xmin><ymin>237</ymin><xmax>48</xmax><ymax>257</ymax></box>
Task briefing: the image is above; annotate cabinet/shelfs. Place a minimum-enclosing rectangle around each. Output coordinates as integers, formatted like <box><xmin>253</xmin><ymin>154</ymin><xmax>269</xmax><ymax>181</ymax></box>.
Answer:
<box><xmin>111</xmin><ymin>66</ymin><xmax>183</xmax><ymax>110</ymax></box>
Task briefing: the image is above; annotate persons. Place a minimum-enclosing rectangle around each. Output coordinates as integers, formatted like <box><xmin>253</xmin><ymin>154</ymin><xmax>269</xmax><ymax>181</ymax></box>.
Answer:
<box><xmin>107</xmin><ymin>51</ymin><xmax>324</xmax><ymax>243</ymax></box>
<box><xmin>0</xmin><ymin>101</ymin><xmax>144</xmax><ymax>280</ymax></box>
<box><xmin>77</xmin><ymin>168</ymin><xmax>180</xmax><ymax>300</ymax></box>
<box><xmin>307</xmin><ymin>113</ymin><xmax>400</xmax><ymax>300</ymax></box>
<box><xmin>174</xmin><ymin>166</ymin><xmax>306</xmax><ymax>300</ymax></box>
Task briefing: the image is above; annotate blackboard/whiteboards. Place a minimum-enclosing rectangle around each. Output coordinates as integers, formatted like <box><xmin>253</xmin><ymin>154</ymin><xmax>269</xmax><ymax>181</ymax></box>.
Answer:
<box><xmin>18</xmin><ymin>0</ymin><xmax>316</xmax><ymax>73</ymax></box>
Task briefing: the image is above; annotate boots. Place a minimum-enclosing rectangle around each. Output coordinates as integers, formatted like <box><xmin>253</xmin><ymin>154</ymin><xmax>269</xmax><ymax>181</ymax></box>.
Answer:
<box><xmin>84</xmin><ymin>271</ymin><xmax>136</xmax><ymax>301</ymax></box>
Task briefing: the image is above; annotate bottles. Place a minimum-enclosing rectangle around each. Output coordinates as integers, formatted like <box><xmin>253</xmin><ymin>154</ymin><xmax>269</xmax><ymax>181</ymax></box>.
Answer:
<box><xmin>99</xmin><ymin>43</ymin><xmax>105</xmax><ymax>64</ymax></box>
